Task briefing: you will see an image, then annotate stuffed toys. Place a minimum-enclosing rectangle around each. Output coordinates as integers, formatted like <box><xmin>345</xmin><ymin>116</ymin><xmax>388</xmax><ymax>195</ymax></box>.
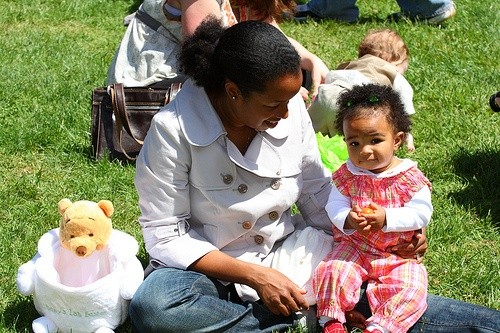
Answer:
<box><xmin>14</xmin><ymin>198</ymin><xmax>145</xmax><ymax>333</ymax></box>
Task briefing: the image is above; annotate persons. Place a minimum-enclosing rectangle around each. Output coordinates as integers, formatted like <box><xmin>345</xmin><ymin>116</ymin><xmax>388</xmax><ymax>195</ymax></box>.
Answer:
<box><xmin>293</xmin><ymin>0</ymin><xmax>456</xmax><ymax>26</ymax></box>
<box><xmin>128</xmin><ymin>20</ymin><xmax>500</xmax><ymax>333</ymax></box>
<box><xmin>307</xmin><ymin>28</ymin><xmax>416</xmax><ymax>152</ymax></box>
<box><xmin>105</xmin><ymin>0</ymin><xmax>330</xmax><ymax>111</ymax></box>
<box><xmin>312</xmin><ymin>83</ymin><xmax>433</xmax><ymax>333</ymax></box>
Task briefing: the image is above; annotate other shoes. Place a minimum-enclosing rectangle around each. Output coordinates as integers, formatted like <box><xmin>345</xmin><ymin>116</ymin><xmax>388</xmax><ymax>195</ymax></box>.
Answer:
<box><xmin>388</xmin><ymin>1</ymin><xmax>456</xmax><ymax>24</ymax></box>
<box><xmin>323</xmin><ymin>320</ymin><xmax>347</xmax><ymax>333</ymax></box>
<box><xmin>364</xmin><ymin>325</ymin><xmax>385</xmax><ymax>333</ymax></box>
<box><xmin>283</xmin><ymin>3</ymin><xmax>359</xmax><ymax>24</ymax></box>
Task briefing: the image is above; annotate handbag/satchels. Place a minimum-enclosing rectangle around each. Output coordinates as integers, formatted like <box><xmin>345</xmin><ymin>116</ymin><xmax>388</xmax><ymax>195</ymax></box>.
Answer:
<box><xmin>90</xmin><ymin>82</ymin><xmax>182</xmax><ymax>158</ymax></box>
